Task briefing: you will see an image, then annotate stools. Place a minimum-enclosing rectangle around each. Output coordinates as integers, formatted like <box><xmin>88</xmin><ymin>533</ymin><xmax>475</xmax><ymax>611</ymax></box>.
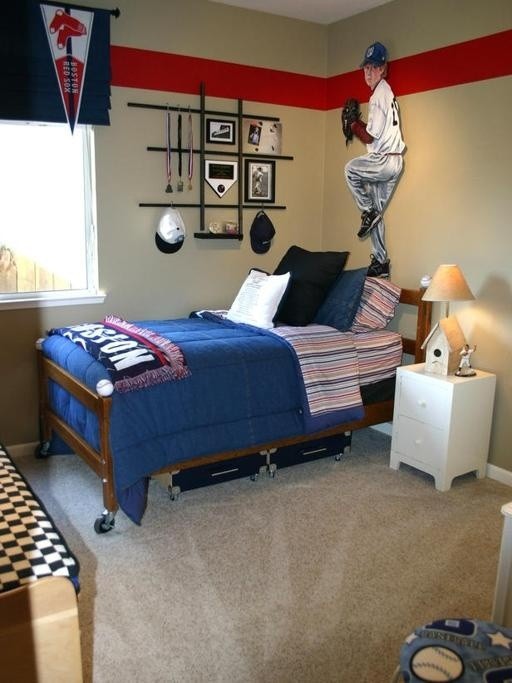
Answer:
<box><xmin>395</xmin><ymin>614</ymin><xmax>511</xmax><ymax>682</ymax></box>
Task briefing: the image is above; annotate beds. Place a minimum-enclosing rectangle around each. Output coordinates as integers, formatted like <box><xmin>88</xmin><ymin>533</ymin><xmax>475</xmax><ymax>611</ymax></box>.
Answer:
<box><xmin>35</xmin><ymin>285</ymin><xmax>435</xmax><ymax>535</ymax></box>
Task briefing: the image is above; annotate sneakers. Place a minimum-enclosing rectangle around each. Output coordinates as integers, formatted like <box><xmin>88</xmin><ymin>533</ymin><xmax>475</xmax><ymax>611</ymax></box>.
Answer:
<box><xmin>367</xmin><ymin>258</ymin><xmax>389</xmax><ymax>278</ymax></box>
<box><xmin>358</xmin><ymin>210</ymin><xmax>382</xmax><ymax>238</ymax></box>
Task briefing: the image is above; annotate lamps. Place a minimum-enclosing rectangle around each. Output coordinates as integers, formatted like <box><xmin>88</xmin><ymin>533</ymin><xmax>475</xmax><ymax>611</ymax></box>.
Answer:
<box><xmin>420</xmin><ymin>262</ymin><xmax>477</xmax><ymax>318</ymax></box>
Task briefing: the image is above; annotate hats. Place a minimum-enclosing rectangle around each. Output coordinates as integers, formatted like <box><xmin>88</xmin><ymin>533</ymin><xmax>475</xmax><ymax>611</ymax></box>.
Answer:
<box><xmin>155</xmin><ymin>207</ymin><xmax>185</xmax><ymax>253</ymax></box>
<box><xmin>251</xmin><ymin>210</ymin><xmax>275</xmax><ymax>254</ymax></box>
<box><xmin>359</xmin><ymin>42</ymin><xmax>387</xmax><ymax>66</ymax></box>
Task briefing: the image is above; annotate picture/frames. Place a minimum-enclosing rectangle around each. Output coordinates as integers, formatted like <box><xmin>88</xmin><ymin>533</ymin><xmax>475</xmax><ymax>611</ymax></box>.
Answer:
<box><xmin>243</xmin><ymin>158</ymin><xmax>277</xmax><ymax>203</ymax></box>
<box><xmin>205</xmin><ymin>118</ymin><xmax>237</xmax><ymax>146</ymax></box>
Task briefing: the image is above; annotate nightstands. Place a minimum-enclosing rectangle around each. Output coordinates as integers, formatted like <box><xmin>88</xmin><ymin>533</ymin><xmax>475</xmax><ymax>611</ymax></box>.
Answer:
<box><xmin>388</xmin><ymin>362</ymin><xmax>498</xmax><ymax>493</ymax></box>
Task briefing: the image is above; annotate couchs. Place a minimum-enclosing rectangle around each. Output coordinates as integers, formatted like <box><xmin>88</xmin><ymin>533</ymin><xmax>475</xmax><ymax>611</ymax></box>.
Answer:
<box><xmin>0</xmin><ymin>438</ymin><xmax>88</xmax><ymax>682</ymax></box>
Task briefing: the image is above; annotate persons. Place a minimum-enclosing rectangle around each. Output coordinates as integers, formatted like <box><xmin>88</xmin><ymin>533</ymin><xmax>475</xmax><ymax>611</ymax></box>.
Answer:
<box><xmin>342</xmin><ymin>43</ymin><xmax>406</xmax><ymax>277</ymax></box>
<box><xmin>252</xmin><ymin>167</ymin><xmax>264</xmax><ymax>196</ymax></box>
<box><xmin>455</xmin><ymin>344</ymin><xmax>477</xmax><ymax>376</ymax></box>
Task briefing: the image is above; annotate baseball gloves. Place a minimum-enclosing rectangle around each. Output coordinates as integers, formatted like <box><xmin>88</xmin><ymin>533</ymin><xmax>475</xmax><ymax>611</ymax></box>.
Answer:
<box><xmin>341</xmin><ymin>98</ymin><xmax>360</xmax><ymax>141</ymax></box>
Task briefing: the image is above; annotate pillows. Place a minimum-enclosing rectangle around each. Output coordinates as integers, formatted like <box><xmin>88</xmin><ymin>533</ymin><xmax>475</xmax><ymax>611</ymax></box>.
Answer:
<box><xmin>349</xmin><ymin>274</ymin><xmax>403</xmax><ymax>333</ymax></box>
<box><xmin>312</xmin><ymin>265</ymin><xmax>369</xmax><ymax>333</ymax></box>
<box><xmin>228</xmin><ymin>267</ymin><xmax>292</xmax><ymax>330</ymax></box>
<box><xmin>272</xmin><ymin>245</ymin><xmax>352</xmax><ymax>329</ymax></box>
